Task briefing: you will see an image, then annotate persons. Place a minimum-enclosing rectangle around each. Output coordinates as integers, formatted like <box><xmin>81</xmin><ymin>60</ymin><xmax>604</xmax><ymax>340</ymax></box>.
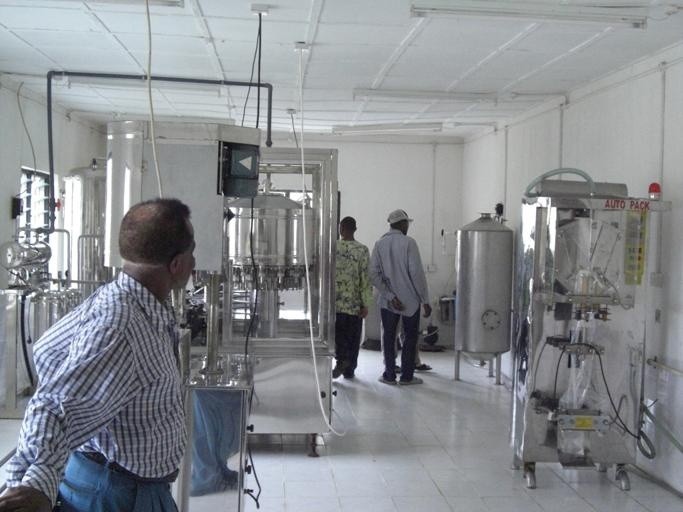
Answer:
<box><xmin>363</xmin><ymin>209</ymin><xmax>431</xmax><ymax>387</ymax></box>
<box><xmin>380</xmin><ymin>304</ymin><xmax>432</xmax><ymax>375</ymax></box>
<box><xmin>331</xmin><ymin>215</ymin><xmax>372</xmax><ymax>381</ymax></box>
<box><xmin>0</xmin><ymin>195</ymin><xmax>199</xmax><ymax>512</ymax></box>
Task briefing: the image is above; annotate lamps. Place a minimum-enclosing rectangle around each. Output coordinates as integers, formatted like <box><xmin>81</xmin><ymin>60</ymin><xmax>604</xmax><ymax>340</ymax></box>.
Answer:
<box><xmin>406</xmin><ymin>4</ymin><xmax>648</xmax><ymax>32</ymax></box>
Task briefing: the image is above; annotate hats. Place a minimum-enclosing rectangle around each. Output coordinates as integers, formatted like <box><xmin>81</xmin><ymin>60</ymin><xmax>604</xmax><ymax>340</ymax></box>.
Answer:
<box><xmin>387</xmin><ymin>209</ymin><xmax>414</xmax><ymax>224</ymax></box>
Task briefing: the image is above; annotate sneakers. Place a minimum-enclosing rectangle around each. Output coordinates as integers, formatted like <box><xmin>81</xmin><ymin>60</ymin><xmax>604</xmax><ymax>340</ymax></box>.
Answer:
<box><xmin>378</xmin><ymin>364</ymin><xmax>431</xmax><ymax>385</ymax></box>
<box><xmin>190</xmin><ymin>469</ymin><xmax>237</xmax><ymax>496</ymax></box>
<box><xmin>333</xmin><ymin>361</ymin><xmax>354</xmax><ymax>378</ymax></box>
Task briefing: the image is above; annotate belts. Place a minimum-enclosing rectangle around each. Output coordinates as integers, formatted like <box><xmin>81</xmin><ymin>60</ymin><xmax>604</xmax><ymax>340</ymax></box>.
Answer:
<box><xmin>83</xmin><ymin>452</ymin><xmax>179</xmax><ymax>483</ymax></box>
